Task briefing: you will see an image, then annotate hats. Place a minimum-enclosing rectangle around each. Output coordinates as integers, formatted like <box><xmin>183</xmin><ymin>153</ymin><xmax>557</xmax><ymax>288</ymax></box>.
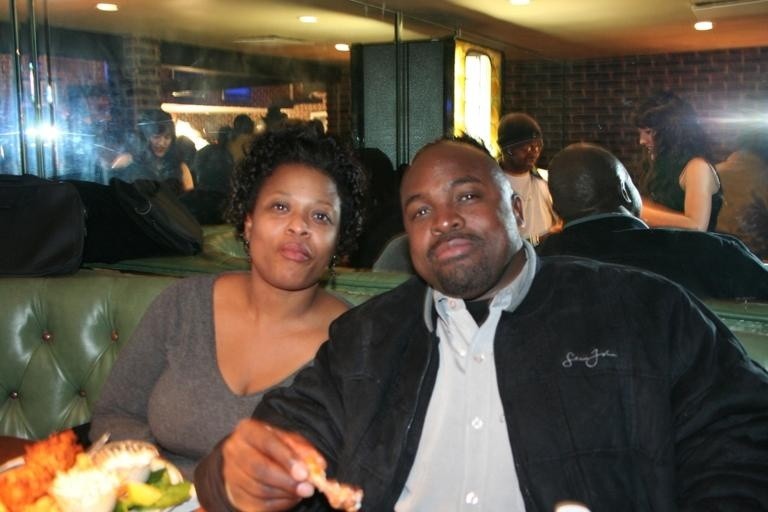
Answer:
<box><xmin>497</xmin><ymin>113</ymin><xmax>542</xmax><ymax>151</ymax></box>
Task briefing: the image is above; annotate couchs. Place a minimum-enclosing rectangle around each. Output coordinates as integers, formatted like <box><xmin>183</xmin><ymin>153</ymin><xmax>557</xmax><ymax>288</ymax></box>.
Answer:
<box><xmin>0</xmin><ymin>270</ymin><xmax>766</xmax><ymax>442</ymax></box>
<box><xmin>86</xmin><ymin>216</ymin><xmax>767</xmax><ymax>330</ymax></box>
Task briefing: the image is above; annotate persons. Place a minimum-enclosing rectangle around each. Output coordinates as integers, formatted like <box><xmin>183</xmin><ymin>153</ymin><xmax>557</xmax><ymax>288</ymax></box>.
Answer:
<box><xmin>106</xmin><ymin>90</ymin><xmax>412</xmax><ymax>296</ymax></box>
<box><xmin>714</xmin><ymin>120</ymin><xmax>768</xmax><ymax>261</ymax></box>
<box><xmin>631</xmin><ymin>91</ymin><xmax>725</xmax><ymax>235</ymax></box>
<box><xmin>534</xmin><ymin>144</ymin><xmax>766</xmax><ymax>295</ymax></box>
<box><xmin>66</xmin><ymin>120</ymin><xmax>369</xmax><ymax>484</ymax></box>
<box><xmin>492</xmin><ymin>114</ymin><xmax>562</xmax><ymax>247</ymax></box>
<box><xmin>196</xmin><ymin>139</ymin><xmax>768</xmax><ymax>512</ymax></box>
